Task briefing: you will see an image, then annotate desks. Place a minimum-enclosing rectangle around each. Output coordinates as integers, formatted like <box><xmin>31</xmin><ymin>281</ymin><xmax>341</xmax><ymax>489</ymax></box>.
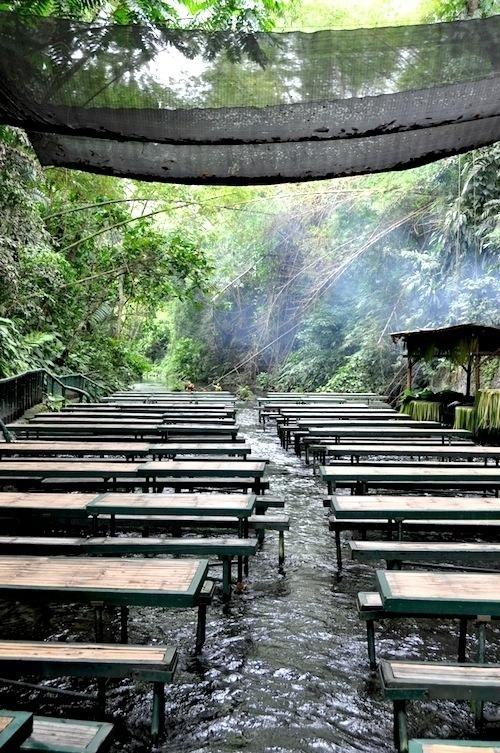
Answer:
<box><xmin>0</xmin><ymin>553</ymin><xmax>210</xmax><ymax>609</ymax></box>
<box><xmin>407</xmin><ymin>737</ymin><xmax>500</xmax><ymax>753</ymax></box>
<box><xmin>251</xmin><ymin>390</ymin><xmax>500</xmax><ymax>571</ymax></box>
<box><xmin>374</xmin><ymin>569</ymin><xmax>500</xmax><ymax>619</ymax></box>
<box><xmin>0</xmin><ymin>707</ymin><xmax>35</xmax><ymax>753</ymax></box>
<box><xmin>0</xmin><ymin>386</ymin><xmax>291</xmax><ymax>601</ymax></box>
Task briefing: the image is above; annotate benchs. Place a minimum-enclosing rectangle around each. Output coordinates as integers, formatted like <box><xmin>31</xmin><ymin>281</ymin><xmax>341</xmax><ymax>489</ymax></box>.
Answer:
<box><xmin>191</xmin><ymin>580</ymin><xmax>218</xmax><ymax>647</ymax></box>
<box><xmin>355</xmin><ymin>590</ymin><xmax>492</xmax><ymax>667</ymax></box>
<box><xmin>15</xmin><ymin>716</ymin><xmax>115</xmax><ymax>753</ymax></box>
<box><xmin>379</xmin><ymin>659</ymin><xmax>500</xmax><ymax>753</ymax></box>
<box><xmin>0</xmin><ymin>638</ymin><xmax>181</xmax><ymax>738</ymax></box>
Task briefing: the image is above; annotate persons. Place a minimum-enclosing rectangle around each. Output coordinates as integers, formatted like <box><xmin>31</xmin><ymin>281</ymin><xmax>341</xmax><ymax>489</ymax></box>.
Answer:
<box><xmin>184</xmin><ymin>381</ymin><xmax>194</xmax><ymax>390</ymax></box>
<box><xmin>212</xmin><ymin>382</ymin><xmax>222</xmax><ymax>390</ymax></box>
<box><xmin>202</xmin><ymin>386</ymin><xmax>208</xmax><ymax>391</ymax></box>
